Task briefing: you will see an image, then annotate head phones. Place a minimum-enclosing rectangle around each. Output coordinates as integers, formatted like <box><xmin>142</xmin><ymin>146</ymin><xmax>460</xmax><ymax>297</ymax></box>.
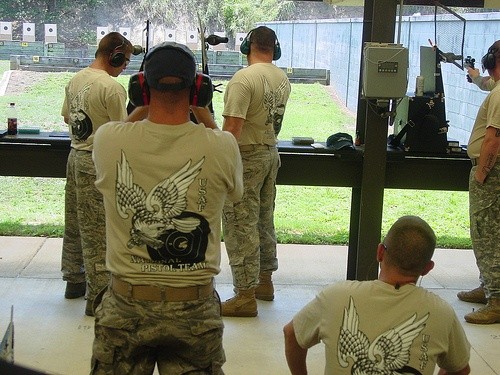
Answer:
<box><xmin>95</xmin><ymin>37</ymin><xmax>131</xmax><ymax>67</ymax></box>
<box><xmin>240</xmin><ymin>26</ymin><xmax>281</xmax><ymax>60</ymax></box>
<box><xmin>128</xmin><ymin>42</ymin><xmax>213</xmax><ymax>109</ymax></box>
<box><xmin>482</xmin><ymin>42</ymin><xmax>496</xmax><ymax>69</ymax></box>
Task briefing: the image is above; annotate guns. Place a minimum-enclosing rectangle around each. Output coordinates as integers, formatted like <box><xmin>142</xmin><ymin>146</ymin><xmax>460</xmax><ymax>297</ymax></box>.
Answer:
<box><xmin>464</xmin><ymin>56</ymin><xmax>476</xmax><ymax>83</ymax></box>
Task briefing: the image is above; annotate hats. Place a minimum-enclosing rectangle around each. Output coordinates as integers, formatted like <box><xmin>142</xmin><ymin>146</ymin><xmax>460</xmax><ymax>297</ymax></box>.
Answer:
<box><xmin>326</xmin><ymin>133</ymin><xmax>355</xmax><ymax>151</ymax></box>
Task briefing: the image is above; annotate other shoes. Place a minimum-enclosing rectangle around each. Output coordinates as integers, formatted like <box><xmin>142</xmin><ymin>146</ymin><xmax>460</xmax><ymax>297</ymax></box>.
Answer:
<box><xmin>65</xmin><ymin>282</ymin><xmax>85</xmax><ymax>299</ymax></box>
<box><xmin>464</xmin><ymin>296</ymin><xmax>500</xmax><ymax>324</ymax></box>
<box><xmin>234</xmin><ymin>272</ymin><xmax>274</xmax><ymax>301</ymax></box>
<box><xmin>457</xmin><ymin>284</ymin><xmax>487</xmax><ymax>304</ymax></box>
<box><xmin>220</xmin><ymin>290</ymin><xmax>259</xmax><ymax>317</ymax></box>
<box><xmin>85</xmin><ymin>299</ymin><xmax>94</xmax><ymax>316</ymax></box>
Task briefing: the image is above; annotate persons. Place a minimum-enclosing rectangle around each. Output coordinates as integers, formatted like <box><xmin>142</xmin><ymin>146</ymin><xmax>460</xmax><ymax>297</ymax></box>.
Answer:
<box><xmin>61</xmin><ymin>32</ymin><xmax>132</xmax><ymax>317</ymax></box>
<box><xmin>282</xmin><ymin>215</ymin><xmax>471</xmax><ymax>375</ymax></box>
<box><xmin>221</xmin><ymin>26</ymin><xmax>291</xmax><ymax>319</ymax></box>
<box><xmin>90</xmin><ymin>42</ymin><xmax>243</xmax><ymax>375</ymax></box>
<box><xmin>457</xmin><ymin>40</ymin><xmax>500</xmax><ymax>324</ymax></box>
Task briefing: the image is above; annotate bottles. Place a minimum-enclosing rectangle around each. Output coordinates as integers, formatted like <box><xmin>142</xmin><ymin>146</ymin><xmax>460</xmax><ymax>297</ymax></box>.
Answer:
<box><xmin>7</xmin><ymin>103</ymin><xmax>17</xmax><ymax>135</ymax></box>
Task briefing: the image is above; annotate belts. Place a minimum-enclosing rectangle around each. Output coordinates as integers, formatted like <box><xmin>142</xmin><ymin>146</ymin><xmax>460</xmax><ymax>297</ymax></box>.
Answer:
<box><xmin>471</xmin><ymin>156</ymin><xmax>500</xmax><ymax>165</ymax></box>
<box><xmin>109</xmin><ymin>275</ymin><xmax>214</xmax><ymax>302</ymax></box>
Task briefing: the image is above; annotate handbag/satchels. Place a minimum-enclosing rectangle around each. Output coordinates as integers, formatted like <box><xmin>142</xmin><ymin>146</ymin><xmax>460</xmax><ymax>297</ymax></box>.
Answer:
<box><xmin>389</xmin><ymin>114</ymin><xmax>443</xmax><ymax>153</ymax></box>
